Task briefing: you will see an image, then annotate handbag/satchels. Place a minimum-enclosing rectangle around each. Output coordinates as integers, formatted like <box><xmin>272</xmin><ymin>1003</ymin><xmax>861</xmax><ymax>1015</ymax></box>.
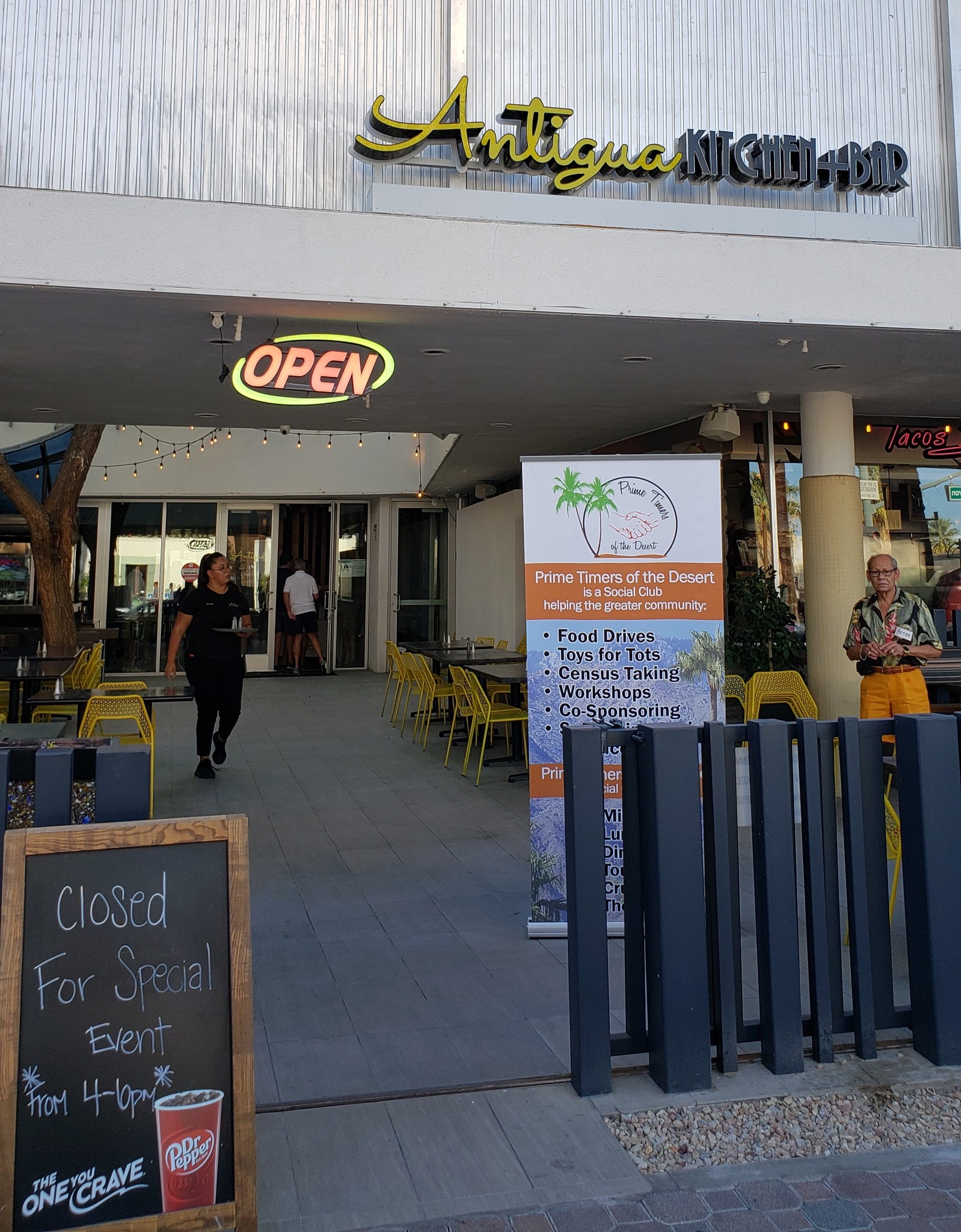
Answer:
<box><xmin>856</xmin><ymin>661</ymin><xmax>874</xmax><ymax>676</ymax></box>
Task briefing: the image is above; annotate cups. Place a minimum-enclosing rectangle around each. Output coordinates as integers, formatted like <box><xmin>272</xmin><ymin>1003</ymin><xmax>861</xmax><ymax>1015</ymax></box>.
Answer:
<box><xmin>151</xmin><ymin>1089</ymin><xmax>224</xmax><ymax>1213</ymax></box>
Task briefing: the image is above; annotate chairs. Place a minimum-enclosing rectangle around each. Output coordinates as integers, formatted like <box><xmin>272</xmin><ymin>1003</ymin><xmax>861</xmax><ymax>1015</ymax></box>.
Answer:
<box><xmin>844</xmin><ymin>743</ymin><xmax>902</xmax><ymax>946</ymax></box>
<box><xmin>0</xmin><ymin>643</ymin><xmax>155</xmax><ymax>819</ymax></box>
<box><xmin>726</xmin><ymin>670</ymin><xmax>840</xmax><ymax>788</ymax></box>
<box><xmin>381</xmin><ymin>632</ymin><xmax>529</xmax><ymax>787</ymax></box>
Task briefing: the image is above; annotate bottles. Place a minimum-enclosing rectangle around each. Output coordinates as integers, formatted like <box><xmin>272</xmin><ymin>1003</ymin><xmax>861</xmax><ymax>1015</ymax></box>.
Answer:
<box><xmin>237</xmin><ymin>616</ymin><xmax>244</xmax><ymax>630</ymax></box>
<box><xmin>17</xmin><ymin>656</ymin><xmax>23</xmax><ymax>670</ymax></box>
<box><xmin>36</xmin><ymin>641</ymin><xmax>42</xmax><ymax>653</ymax></box>
<box><xmin>443</xmin><ymin>632</ymin><xmax>446</xmax><ymax>644</ymax></box>
<box><xmin>472</xmin><ymin>641</ymin><xmax>475</xmax><ymax>653</ymax></box>
<box><xmin>467</xmin><ymin>641</ymin><xmax>470</xmax><ymax>653</ymax></box>
<box><xmin>232</xmin><ymin>617</ymin><xmax>237</xmax><ymax>630</ymax></box>
<box><xmin>23</xmin><ymin>656</ymin><xmax>27</xmax><ymax>671</ymax></box>
<box><xmin>42</xmin><ymin>641</ymin><xmax>47</xmax><ymax>653</ymax></box>
<box><xmin>53</xmin><ymin>679</ymin><xmax>62</xmax><ymax>696</ymax></box>
<box><xmin>60</xmin><ymin>677</ymin><xmax>65</xmax><ymax>694</ymax></box>
<box><xmin>447</xmin><ymin>633</ymin><xmax>451</xmax><ymax>644</ymax></box>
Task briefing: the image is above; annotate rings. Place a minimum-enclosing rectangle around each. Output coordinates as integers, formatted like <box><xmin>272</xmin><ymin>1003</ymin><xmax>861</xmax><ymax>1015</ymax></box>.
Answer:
<box><xmin>868</xmin><ymin>651</ymin><xmax>871</xmax><ymax>655</ymax></box>
<box><xmin>890</xmin><ymin>649</ymin><xmax>894</xmax><ymax>654</ymax></box>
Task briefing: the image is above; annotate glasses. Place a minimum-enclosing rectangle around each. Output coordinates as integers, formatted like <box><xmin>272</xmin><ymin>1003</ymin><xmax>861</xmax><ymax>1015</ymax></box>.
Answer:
<box><xmin>212</xmin><ymin>564</ymin><xmax>232</xmax><ymax>573</ymax></box>
<box><xmin>868</xmin><ymin>568</ymin><xmax>897</xmax><ymax>577</ymax></box>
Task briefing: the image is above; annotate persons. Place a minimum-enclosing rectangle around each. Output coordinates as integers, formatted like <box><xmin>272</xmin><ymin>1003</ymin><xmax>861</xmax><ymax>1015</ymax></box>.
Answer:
<box><xmin>275</xmin><ymin>553</ymin><xmax>361</xmax><ymax>676</ymax></box>
<box><xmin>398</xmin><ymin>559</ymin><xmax>422</xmax><ymax>640</ymax></box>
<box><xmin>163</xmin><ymin>552</ymin><xmax>255</xmax><ymax>779</ymax></box>
<box><xmin>842</xmin><ymin>554</ymin><xmax>944</xmax><ymax>744</ymax></box>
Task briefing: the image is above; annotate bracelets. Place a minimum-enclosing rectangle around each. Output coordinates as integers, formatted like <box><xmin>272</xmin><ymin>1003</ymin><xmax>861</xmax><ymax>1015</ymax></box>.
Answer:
<box><xmin>859</xmin><ymin>644</ymin><xmax>868</xmax><ymax>661</ymax></box>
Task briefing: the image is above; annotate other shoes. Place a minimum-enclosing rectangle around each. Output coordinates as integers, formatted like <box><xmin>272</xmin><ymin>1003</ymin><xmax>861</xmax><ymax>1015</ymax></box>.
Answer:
<box><xmin>275</xmin><ymin>662</ymin><xmax>283</xmax><ymax>668</ymax></box>
<box><xmin>286</xmin><ymin>662</ymin><xmax>294</xmax><ymax>670</ymax></box>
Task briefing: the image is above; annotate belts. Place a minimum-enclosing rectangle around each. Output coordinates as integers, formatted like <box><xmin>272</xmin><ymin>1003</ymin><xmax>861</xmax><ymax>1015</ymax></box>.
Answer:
<box><xmin>873</xmin><ymin>666</ymin><xmax>920</xmax><ymax>675</ymax></box>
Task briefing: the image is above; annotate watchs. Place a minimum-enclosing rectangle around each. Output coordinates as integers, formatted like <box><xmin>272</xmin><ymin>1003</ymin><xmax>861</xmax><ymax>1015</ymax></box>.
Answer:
<box><xmin>901</xmin><ymin>644</ymin><xmax>910</xmax><ymax>657</ymax></box>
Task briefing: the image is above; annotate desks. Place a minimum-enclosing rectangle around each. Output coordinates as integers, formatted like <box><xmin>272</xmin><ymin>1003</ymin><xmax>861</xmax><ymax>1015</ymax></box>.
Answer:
<box><xmin>462</xmin><ymin>664</ymin><xmax>529</xmax><ymax>783</ymax></box>
<box><xmin>0</xmin><ymin>660</ymin><xmax>75</xmax><ymax>723</ymax></box>
<box><xmin>421</xmin><ymin>649</ymin><xmax>527</xmax><ymax>747</ymax></box>
<box><xmin>25</xmin><ymin>687</ymin><xmax>195</xmax><ymax>738</ymax></box>
<box><xmin>0</xmin><ymin>645</ymin><xmax>76</xmax><ymax>693</ymax></box>
<box><xmin>394</xmin><ymin>640</ymin><xmax>494</xmax><ymax>725</ymax></box>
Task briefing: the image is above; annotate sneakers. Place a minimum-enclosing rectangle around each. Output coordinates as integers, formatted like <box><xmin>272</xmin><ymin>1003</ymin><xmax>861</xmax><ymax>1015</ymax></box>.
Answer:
<box><xmin>212</xmin><ymin>731</ymin><xmax>227</xmax><ymax>765</ymax></box>
<box><xmin>195</xmin><ymin>758</ymin><xmax>224</xmax><ymax>780</ymax></box>
<box><xmin>320</xmin><ymin>658</ymin><xmax>326</xmax><ymax>672</ymax></box>
<box><xmin>292</xmin><ymin>663</ymin><xmax>300</xmax><ymax>673</ymax></box>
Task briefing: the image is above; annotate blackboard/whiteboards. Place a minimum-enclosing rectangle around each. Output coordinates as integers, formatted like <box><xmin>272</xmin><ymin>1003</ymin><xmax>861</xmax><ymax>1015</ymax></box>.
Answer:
<box><xmin>3</xmin><ymin>809</ymin><xmax>255</xmax><ymax>1232</ymax></box>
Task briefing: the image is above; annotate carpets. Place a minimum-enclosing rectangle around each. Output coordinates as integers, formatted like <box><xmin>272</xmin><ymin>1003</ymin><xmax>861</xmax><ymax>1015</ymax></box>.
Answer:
<box><xmin>278</xmin><ymin>656</ymin><xmax>328</xmax><ymax>669</ymax></box>
